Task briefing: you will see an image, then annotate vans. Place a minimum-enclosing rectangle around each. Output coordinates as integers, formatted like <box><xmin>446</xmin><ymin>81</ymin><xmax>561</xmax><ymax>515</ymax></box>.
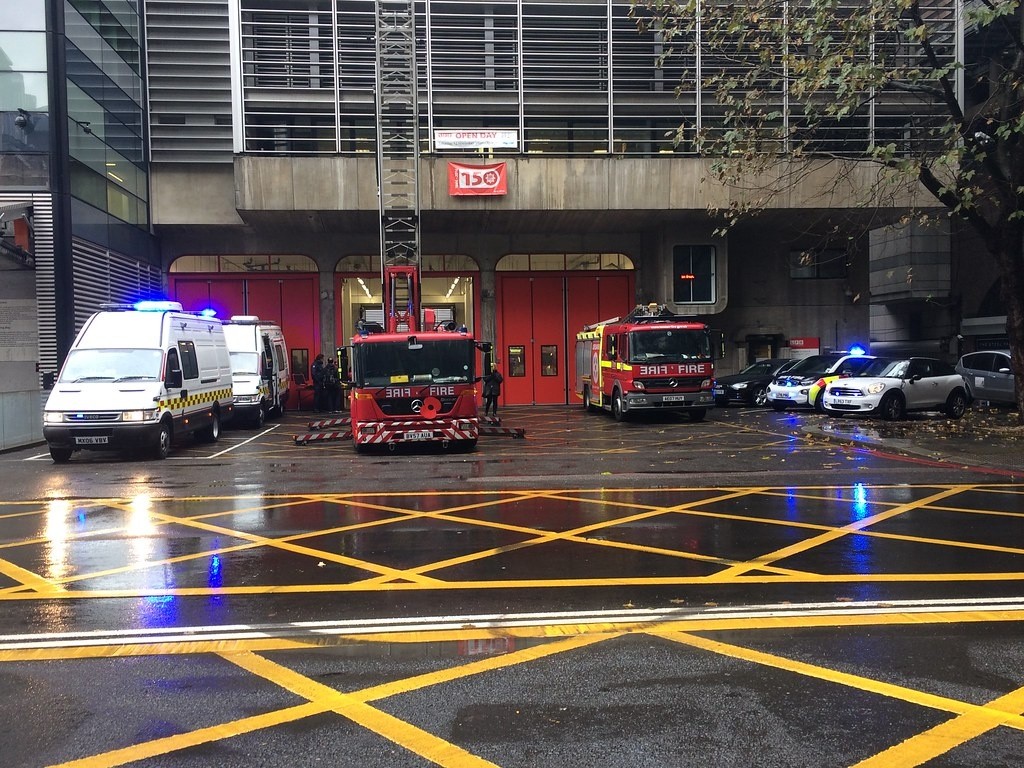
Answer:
<box><xmin>218</xmin><ymin>316</ymin><xmax>291</xmax><ymax>430</ymax></box>
<box><xmin>44</xmin><ymin>301</ymin><xmax>234</xmax><ymax>464</ymax></box>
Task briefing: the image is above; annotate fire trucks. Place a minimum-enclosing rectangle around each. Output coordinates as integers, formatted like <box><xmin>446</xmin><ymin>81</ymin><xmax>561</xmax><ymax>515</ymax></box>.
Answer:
<box><xmin>574</xmin><ymin>303</ymin><xmax>726</xmax><ymax>422</ymax></box>
<box><xmin>294</xmin><ymin>1</ymin><xmax>525</xmax><ymax>459</ymax></box>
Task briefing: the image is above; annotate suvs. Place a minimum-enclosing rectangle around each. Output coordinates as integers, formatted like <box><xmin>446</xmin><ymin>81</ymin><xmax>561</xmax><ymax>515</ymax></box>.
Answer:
<box><xmin>822</xmin><ymin>356</ymin><xmax>970</xmax><ymax>427</ymax></box>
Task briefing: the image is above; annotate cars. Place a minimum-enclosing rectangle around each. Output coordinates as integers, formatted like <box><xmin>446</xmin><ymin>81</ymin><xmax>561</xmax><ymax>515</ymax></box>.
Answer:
<box><xmin>765</xmin><ymin>353</ymin><xmax>877</xmax><ymax>414</ymax></box>
<box><xmin>954</xmin><ymin>350</ymin><xmax>1016</xmax><ymax>407</ymax></box>
<box><xmin>713</xmin><ymin>357</ymin><xmax>803</xmax><ymax>409</ymax></box>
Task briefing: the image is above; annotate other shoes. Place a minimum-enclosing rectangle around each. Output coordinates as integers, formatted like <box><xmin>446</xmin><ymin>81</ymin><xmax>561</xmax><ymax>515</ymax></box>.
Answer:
<box><xmin>493</xmin><ymin>413</ymin><xmax>496</xmax><ymax>416</ymax></box>
<box><xmin>320</xmin><ymin>409</ymin><xmax>325</xmax><ymax>411</ymax></box>
<box><xmin>329</xmin><ymin>410</ymin><xmax>332</xmax><ymax>413</ymax></box>
<box><xmin>485</xmin><ymin>412</ymin><xmax>488</xmax><ymax>416</ymax></box>
<box><xmin>312</xmin><ymin>408</ymin><xmax>320</xmax><ymax>413</ymax></box>
<box><xmin>333</xmin><ymin>409</ymin><xmax>342</xmax><ymax>412</ymax></box>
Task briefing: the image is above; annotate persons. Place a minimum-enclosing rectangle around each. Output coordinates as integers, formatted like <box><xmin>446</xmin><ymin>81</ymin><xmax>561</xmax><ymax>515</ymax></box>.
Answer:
<box><xmin>320</xmin><ymin>357</ymin><xmax>343</xmax><ymax>414</ymax></box>
<box><xmin>310</xmin><ymin>353</ymin><xmax>325</xmax><ymax>413</ymax></box>
<box><xmin>481</xmin><ymin>362</ymin><xmax>503</xmax><ymax>416</ymax></box>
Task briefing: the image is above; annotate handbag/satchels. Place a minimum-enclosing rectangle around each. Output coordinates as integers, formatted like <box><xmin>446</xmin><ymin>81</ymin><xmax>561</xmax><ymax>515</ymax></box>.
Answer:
<box><xmin>482</xmin><ymin>378</ymin><xmax>491</xmax><ymax>398</ymax></box>
